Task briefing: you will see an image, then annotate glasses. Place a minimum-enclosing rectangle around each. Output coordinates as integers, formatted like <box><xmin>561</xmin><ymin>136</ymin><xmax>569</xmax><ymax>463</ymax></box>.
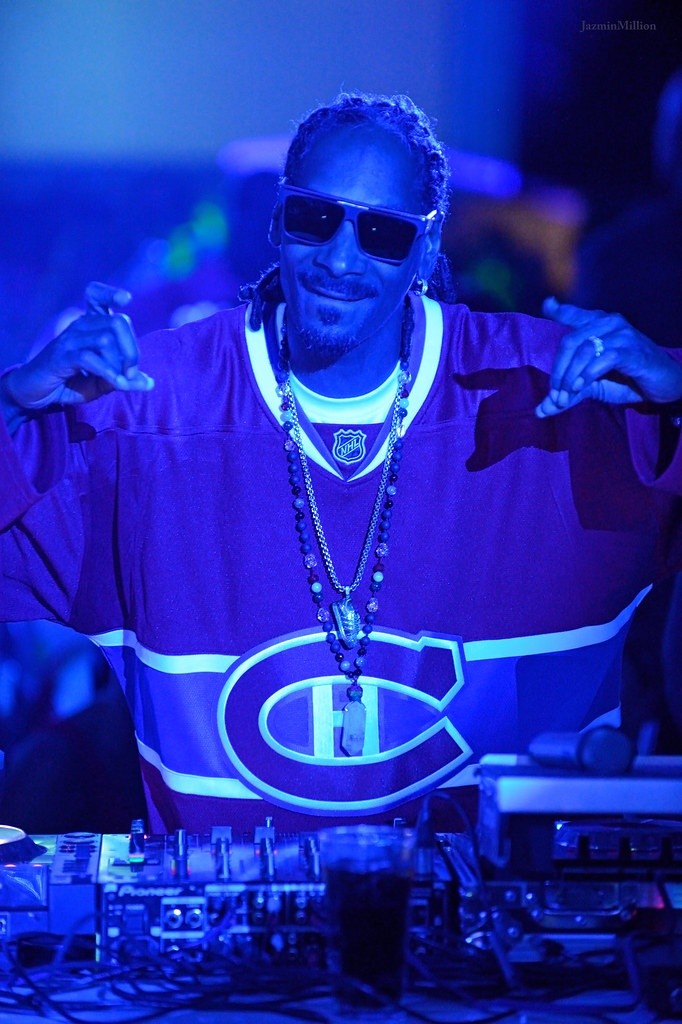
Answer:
<box><xmin>275</xmin><ymin>176</ymin><xmax>436</xmax><ymax>265</ymax></box>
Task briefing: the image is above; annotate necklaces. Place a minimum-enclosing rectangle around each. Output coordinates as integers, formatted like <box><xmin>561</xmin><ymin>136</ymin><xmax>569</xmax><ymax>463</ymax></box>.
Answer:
<box><xmin>272</xmin><ymin>289</ymin><xmax>416</xmax><ymax>755</ymax></box>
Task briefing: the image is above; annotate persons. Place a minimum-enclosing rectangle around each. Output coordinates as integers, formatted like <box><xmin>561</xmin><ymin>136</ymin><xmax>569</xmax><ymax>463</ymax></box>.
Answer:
<box><xmin>1</xmin><ymin>89</ymin><xmax>682</xmax><ymax>852</ymax></box>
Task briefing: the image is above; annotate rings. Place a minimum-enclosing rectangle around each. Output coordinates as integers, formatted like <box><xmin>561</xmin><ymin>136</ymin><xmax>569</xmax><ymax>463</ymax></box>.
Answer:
<box><xmin>589</xmin><ymin>335</ymin><xmax>605</xmax><ymax>357</ymax></box>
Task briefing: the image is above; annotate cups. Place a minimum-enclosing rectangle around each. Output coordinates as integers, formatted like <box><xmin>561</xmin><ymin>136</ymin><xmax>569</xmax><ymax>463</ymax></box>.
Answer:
<box><xmin>322</xmin><ymin>825</ymin><xmax>417</xmax><ymax>1010</ymax></box>
